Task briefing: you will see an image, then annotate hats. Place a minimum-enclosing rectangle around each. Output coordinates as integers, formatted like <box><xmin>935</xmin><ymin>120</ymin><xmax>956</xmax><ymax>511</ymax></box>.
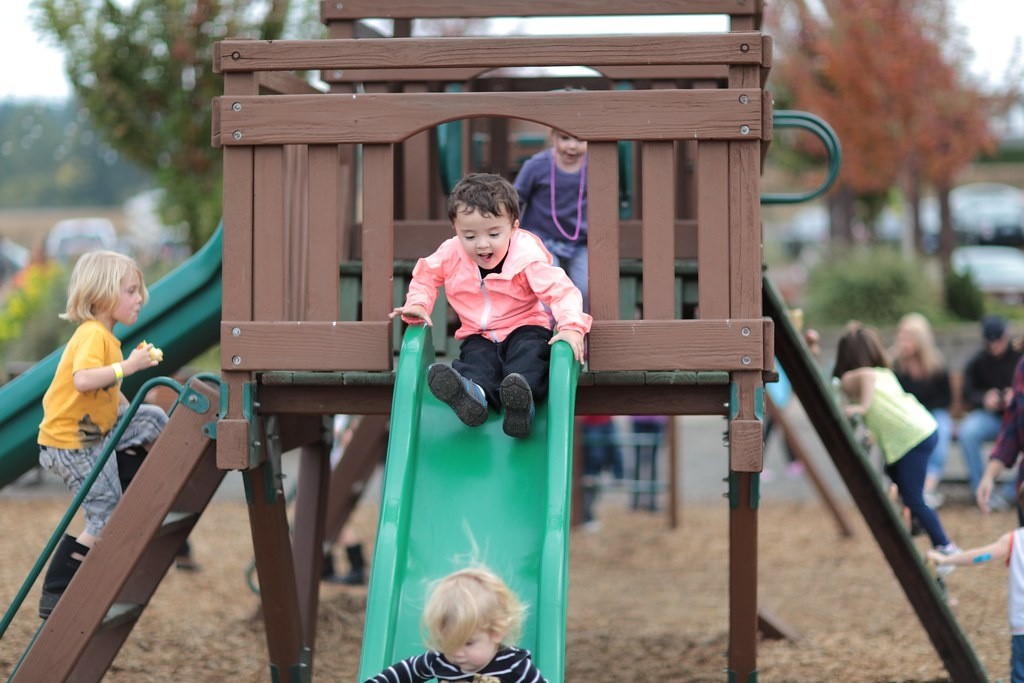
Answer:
<box><xmin>983</xmin><ymin>314</ymin><xmax>1006</xmax><ymax>340</ymax></box>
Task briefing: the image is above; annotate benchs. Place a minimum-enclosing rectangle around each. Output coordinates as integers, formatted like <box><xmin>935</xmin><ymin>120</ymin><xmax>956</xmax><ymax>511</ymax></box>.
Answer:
<box><xmin>937</xmin><ymin>365</ymin><xmax>1019</xmax><ymax>488</ymax></box>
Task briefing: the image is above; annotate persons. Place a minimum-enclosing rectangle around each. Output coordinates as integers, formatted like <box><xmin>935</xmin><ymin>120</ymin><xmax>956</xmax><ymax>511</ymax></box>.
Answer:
<box><xmin>761</xmin><ymin>304</ymin><xmax>815</xmax><ymax>486</ymax></box>
<box><xmin>974</xmin><ymin>335</ymin><xmax>1023</xmax><ymax>518</ymax></box>
<box><xmin>37</xmin><ymin>251</ymin><xmax>172</xmax><ymax>621</ymax></box>
<box><xmin>510</xmin><ymin>124</ymin><xmax>592</xmax><ymax>325</ymax></box>
<box><xmin>388</xmin><ymin>173</ymin><xmax>592</xmax><ymax>439</ymax></box>
<box><xmin>361</xmin><ymin>567</ymin><xmax>551</xmax><ymax>683</ymax></box>
<box><xmin>575</xmin><ymin>411</ymin><xmax>681</xmax><ymax>539</ymax></box>
<box><xmin>316</xmin><ymin>408</ymin><xmax>373</xmax><ymax>584</ymax></box>
<box><xmin>959</xmin><ymin>319</ymin><xmax>1023</xmax><ymax>514</ymax></box>
<box><xmin>927</xmin><ymin>460</ymin><xmax>1024</xmax><ymax>683</ymax></box>
<box><xmin>829</xmin><ymin>320</ymin><xmax>962</xmax><ymax>580</ymax></box>
<box><xmin>882</xmin><ymin>312</ymin><xmax>958</xmax><ymax>532</ymax></box>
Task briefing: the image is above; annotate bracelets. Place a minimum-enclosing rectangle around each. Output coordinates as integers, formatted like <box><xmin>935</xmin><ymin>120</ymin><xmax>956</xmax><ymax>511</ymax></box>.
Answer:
<box><xmin>112</xmin><ymin>362</ymin><xmax>126</xmax><ymax>382</ymax></box>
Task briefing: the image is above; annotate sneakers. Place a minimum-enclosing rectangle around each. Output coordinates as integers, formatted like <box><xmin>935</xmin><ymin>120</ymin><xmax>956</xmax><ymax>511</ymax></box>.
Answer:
<box><xmin>499</xmin><ymin>372</ymin><xmax>535</xmax><ymax>439</ymax></box>
<box><xmin>428</xmin><ymin>363</ymin><xmax>488</xmax><ymax>427</ymax></box>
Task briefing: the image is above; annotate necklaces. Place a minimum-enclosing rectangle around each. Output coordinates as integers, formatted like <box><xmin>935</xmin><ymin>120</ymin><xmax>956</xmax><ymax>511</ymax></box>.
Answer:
<box><xmin>549</xmin><ymin>148</ymin><xmax>586</xmax><ymax>242</ymax></box>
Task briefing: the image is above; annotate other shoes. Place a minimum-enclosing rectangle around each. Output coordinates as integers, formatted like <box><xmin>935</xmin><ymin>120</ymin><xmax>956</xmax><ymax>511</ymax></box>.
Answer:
<box><xmin>339</xmin><ymin>565</ymin><xmax>367</xmax><ymax>585</ymax></box>
<box><xmin>38</xmin><ymin>533</ymin><xmax>93</xmax><ymax>623</ymax></box>
<box><xmin>986</xmin><ymin>494</ymin><xmax>1011</xmax><ymax>513</ymax></box>
<box><xmin>115</xmin><ymin>445</ymin><xmax>148</xmax><ymax>495</ymax></box>
<box><xmin>176</xmin><ymin>554</ymin><xmax>198</xmax><ymax>572</ymax></box>
<box><xmin>921</xmin><ymin>488</ymin><xmax>946</xmax><ymax>511</ymax></box>
<box><xmin>933</xmin><ymin>541</ymin><xmax>963</xmax><ymax>576</ymax></box>
<box><xmin>322</xmin><ymin>573</ymin><xmax>342</xmax><ymax>586</ymax></box>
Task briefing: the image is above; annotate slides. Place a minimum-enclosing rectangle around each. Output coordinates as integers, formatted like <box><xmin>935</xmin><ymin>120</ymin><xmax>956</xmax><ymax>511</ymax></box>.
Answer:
<box><xmin>0</xmin><ymin>214</ymin><xmax>224</xmax><ymax>490</ymax></box>
<box><xmin>358</xmin><ymin>323</ymin><xmax>581</xmax><ymax>683</ymax></box>
